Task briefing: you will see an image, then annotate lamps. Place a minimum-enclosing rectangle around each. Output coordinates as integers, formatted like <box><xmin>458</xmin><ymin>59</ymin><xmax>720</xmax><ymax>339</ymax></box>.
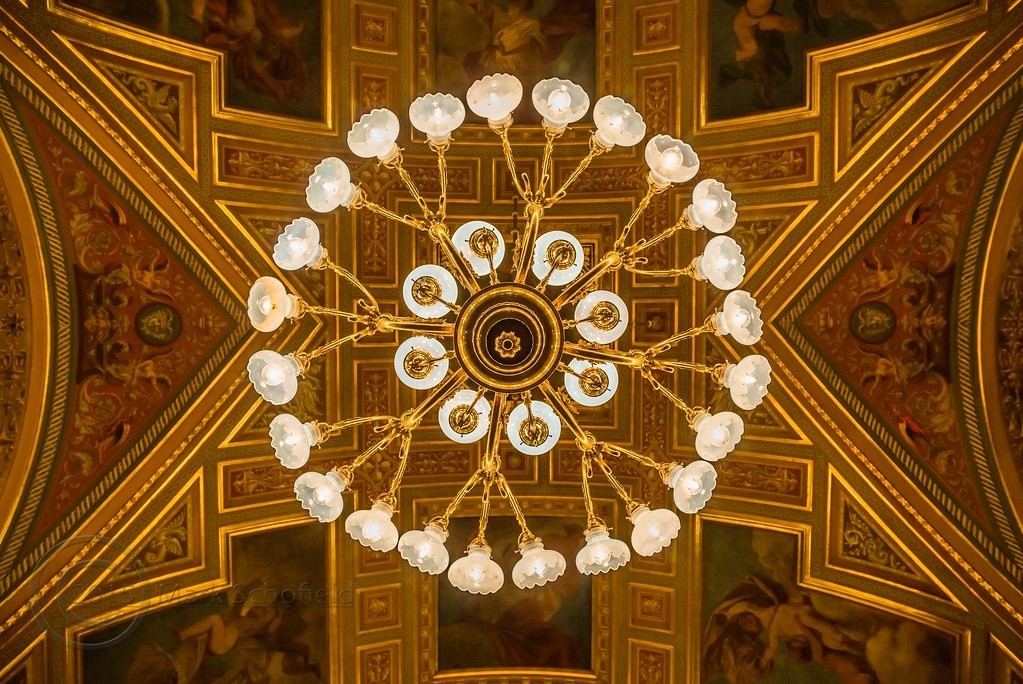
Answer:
<box><xmin>243</xmin><ymin>67</ymin><xmax>774</xmax><ymax>600</ymax></box>
